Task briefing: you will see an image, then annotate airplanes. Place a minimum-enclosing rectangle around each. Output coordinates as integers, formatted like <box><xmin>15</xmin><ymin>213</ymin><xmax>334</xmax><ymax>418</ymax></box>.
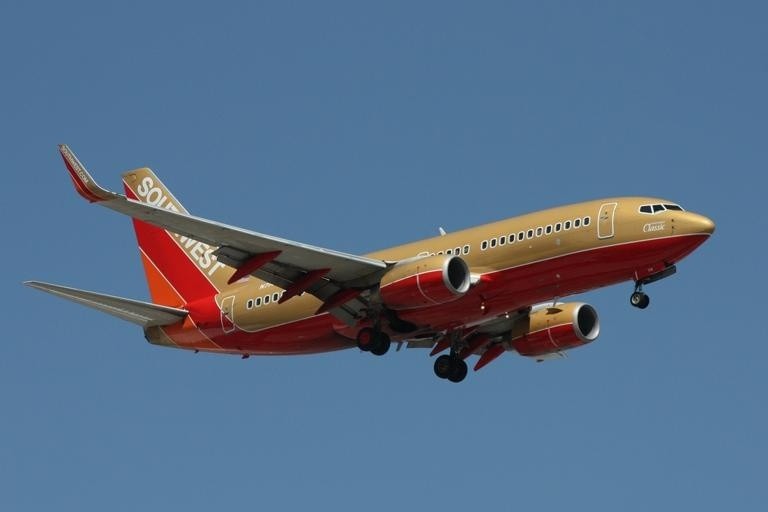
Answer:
<box><xmin>20</xmin><ymin>144</ymin><xmax>716</xmax><ymax>383</ymax></box>
